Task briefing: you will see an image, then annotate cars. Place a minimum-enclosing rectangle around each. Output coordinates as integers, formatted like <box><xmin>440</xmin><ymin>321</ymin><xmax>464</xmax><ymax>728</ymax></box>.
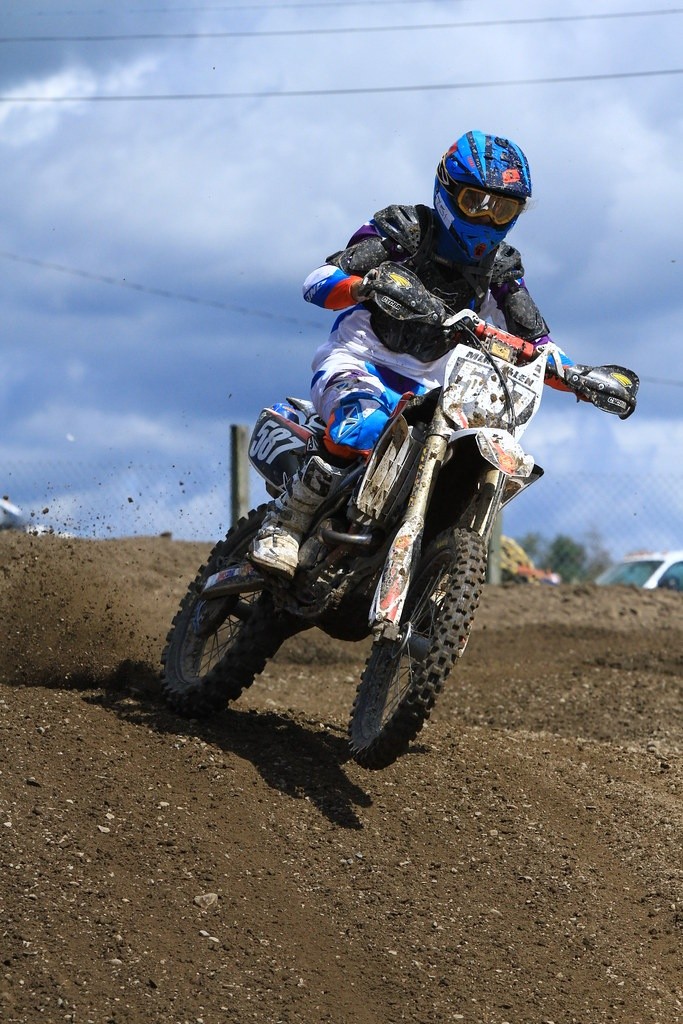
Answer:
<box><xmin>594</xmin><ymin>551</ymin><xmax>683</xmax><ymax>590</ymax></box>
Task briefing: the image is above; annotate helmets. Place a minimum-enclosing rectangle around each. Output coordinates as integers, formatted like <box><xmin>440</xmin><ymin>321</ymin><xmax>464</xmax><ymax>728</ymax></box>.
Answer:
<box><xmin>434</xmin><ymin>131</ymin><xmax>531</xmax><ymax>263</ymax></box>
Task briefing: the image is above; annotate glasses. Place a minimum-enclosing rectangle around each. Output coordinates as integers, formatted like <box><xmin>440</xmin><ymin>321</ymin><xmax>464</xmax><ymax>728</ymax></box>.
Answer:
<box><xmin>437</xmin><ymin>153</ymin><xmax>527</xmax><ymax>225</ymax></box>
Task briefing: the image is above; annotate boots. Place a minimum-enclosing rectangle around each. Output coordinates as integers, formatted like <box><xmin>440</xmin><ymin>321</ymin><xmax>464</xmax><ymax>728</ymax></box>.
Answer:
<box><xmin>249</xmin><ymin>450</ymin><xmax>359</xmax><ymax>578</ymax></box>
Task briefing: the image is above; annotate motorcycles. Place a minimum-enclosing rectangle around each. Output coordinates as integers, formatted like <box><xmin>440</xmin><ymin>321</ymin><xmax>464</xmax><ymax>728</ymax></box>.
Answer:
<box><xmin>159</xmin><ymin>260</ymin><xmax>641</xmax><ymax>771</ymax></box>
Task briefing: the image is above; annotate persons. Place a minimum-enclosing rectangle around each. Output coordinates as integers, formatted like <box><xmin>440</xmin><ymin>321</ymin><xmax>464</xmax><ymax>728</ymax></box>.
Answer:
<box><xmin>246</xmin><ymin>130</ymin><xmax>639</xmax><ymax>580</ymax></box>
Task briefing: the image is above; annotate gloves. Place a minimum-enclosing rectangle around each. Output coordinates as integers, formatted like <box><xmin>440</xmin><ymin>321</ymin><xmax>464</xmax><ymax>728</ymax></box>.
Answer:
<box><xmin>358</xmin><ymin>265</ymin><xmax>383</xmax><ymax>301</ymax></box>
<box><xmin>566</xmin><ymin>362</ymin><xmax>634</xmax><ymax>402</ymax></box>
